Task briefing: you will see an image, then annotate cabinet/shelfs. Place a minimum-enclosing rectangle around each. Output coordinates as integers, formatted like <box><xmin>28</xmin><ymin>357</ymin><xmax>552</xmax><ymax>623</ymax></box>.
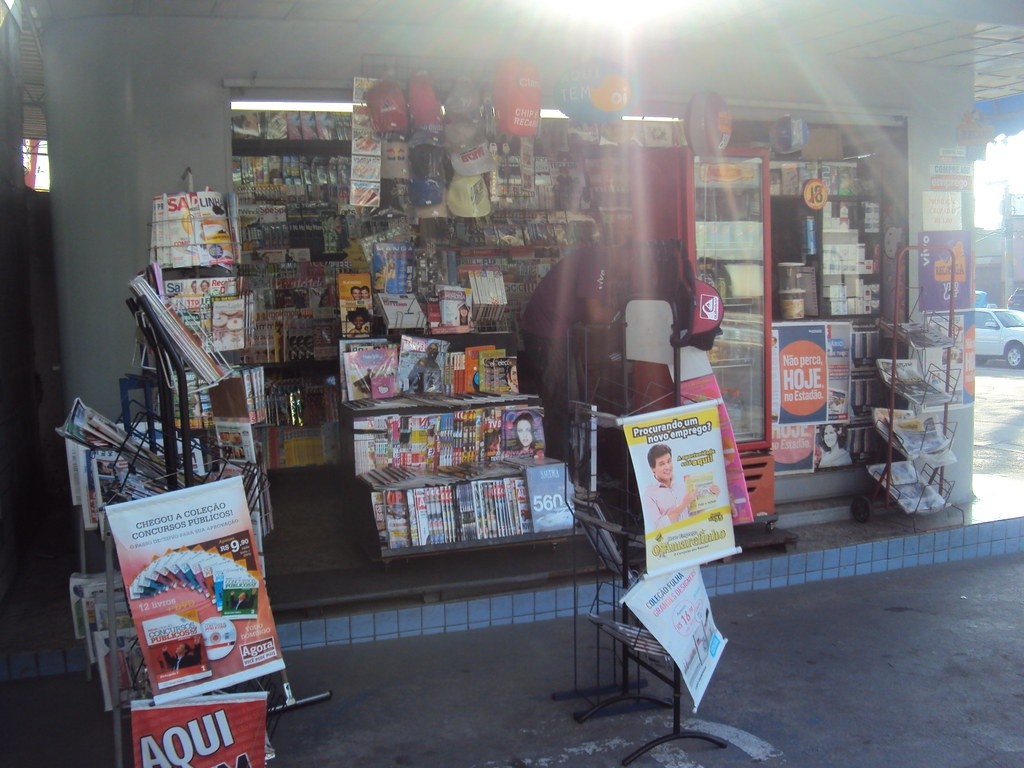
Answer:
<box><xmin>76</xmin><ymin>52</ymin><xmax>962</xmax><ymax>768</ymax></box>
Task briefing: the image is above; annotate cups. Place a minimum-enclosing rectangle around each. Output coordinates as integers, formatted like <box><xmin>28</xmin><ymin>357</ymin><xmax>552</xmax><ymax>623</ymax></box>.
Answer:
<box><xmin>777</xmin><ymin>262</ymin><xmax>806</xmax><ymax>291</ymax></box>
<box><xmin>779</xmin><ymin>288</ymin><xmax>806</xmax><ymax>320</ymax></box>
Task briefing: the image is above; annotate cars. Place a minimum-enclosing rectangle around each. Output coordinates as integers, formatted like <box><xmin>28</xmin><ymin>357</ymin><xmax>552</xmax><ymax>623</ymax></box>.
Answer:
<box><xmin>975</xmin><ymin>307</ymin><xmax>1024</xmax><ymax>369</ymax></box>
<box><xmin>1008</xmin><ymin>287</ymin><xmax>1024</xmax><ymax>312</ymax></box>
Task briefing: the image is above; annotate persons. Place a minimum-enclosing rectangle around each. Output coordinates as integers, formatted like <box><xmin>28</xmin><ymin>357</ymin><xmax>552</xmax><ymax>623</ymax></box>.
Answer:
<box><xmin>161</xmin><ymin>636</ymin><xmax>201</xmax><ymax>670</ymax></box>
<box><xmin>507</xmin><ymin>413</ymin><xmax>543</xmax><ymax>458</ymax></box>
<box><xmin>817</xmin><ymin>424</ymin><xmax>853</xmax><ymax>468</ymax></box>
<box><xmin>230</xmin><ymin>588</ymin><xmax>257</xmax><ymax>611</ymax></box>
<box><xmin>645</xmin><ymin>443</ymin><xmax>721</xmax><ymax>528</ymax></box>
<box><xmin>522</xmin><ymin>241</ymin><xmax>641</xmax><ymax>463</ymax></box>
<box><xmin>347</xmin><ymin>285</ymin><xmax>360</xmax><ymax>304</ymax></box>
<box><xmin>506</xmin><ymin>364</ymin><xmax>519</xmax><ymax>393</ymax></box>
<box><xmin>454</xmin><ymin>303</ymin><xmax>471</xmax><ymax>326</ymax></box>
<box><xmin>361</xmin><ymin>286</ymin><xmax>373</xmax><ymax>314</ymax></box>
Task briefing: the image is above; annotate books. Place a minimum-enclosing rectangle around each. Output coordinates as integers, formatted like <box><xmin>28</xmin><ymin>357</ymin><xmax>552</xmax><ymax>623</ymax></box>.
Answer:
<box><xmin>57</xmin><ymin>188</ymin><xmax>271</xmax><ymax>715</ymax></box>
<box><xmin>337</xmin><ymin>270</ymin><xmax>576</xmax><ymax>549</ymax></box>
